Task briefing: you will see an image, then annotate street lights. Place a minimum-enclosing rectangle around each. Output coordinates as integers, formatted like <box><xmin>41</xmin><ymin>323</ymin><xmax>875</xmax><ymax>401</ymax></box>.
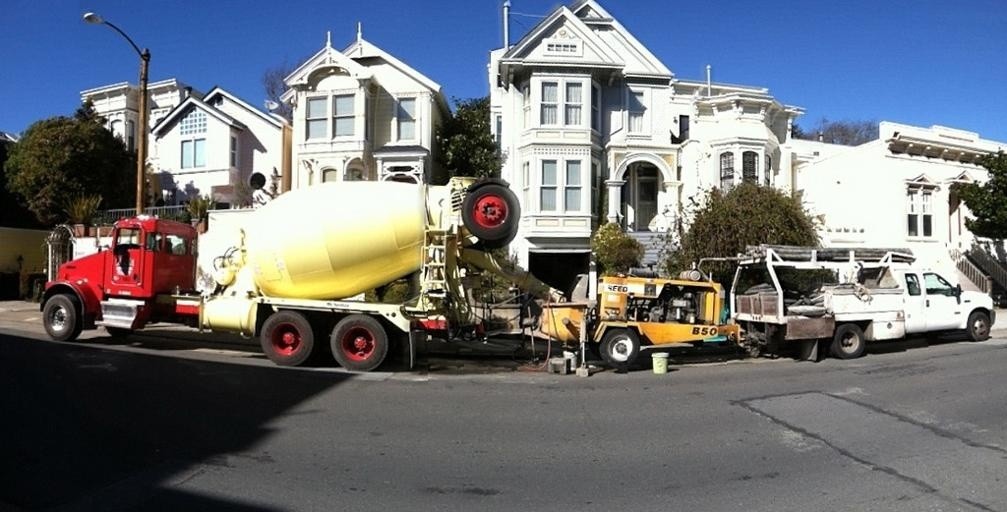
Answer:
<box><xmin>83</xmin><ymin>12</ymin><xmax>151</xmax><ymax>216</ymax></box>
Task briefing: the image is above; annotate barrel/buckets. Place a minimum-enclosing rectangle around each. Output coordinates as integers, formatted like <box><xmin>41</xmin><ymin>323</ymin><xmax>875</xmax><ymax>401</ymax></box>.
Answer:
<box><xmin>563</xmin><ymin>350</ymin><xmax>579</xmax><ymax>372</ymax></box>
<box><xmin>651</xmin><ymin>352</ymin><xmax>669</xmax><ymax>375</ymax></box>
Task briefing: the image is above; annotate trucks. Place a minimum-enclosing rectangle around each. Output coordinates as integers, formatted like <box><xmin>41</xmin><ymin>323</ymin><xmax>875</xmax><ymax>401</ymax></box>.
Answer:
<box><xmin>39</xmin><ymin>172</ymin><xmax>566</xmax><ymax>369</ymax></box>
<box><xmin>537</xmin><ymin>244</ymin><xmax>996</xmax><ymax>364</ymax></box>
